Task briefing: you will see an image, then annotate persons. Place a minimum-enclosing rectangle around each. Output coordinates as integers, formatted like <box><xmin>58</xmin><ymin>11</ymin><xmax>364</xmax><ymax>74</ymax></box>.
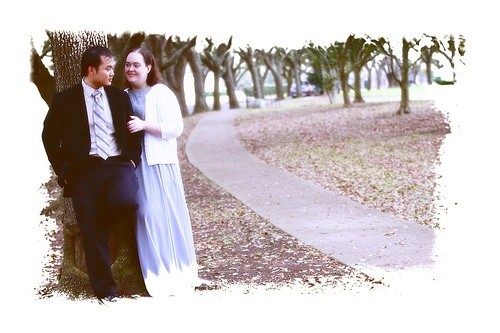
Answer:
<box><xmin>42</xmin><ymin>46</ymin><xmax>141</xmax><ymax>302</ymax></box>
<box><xmin>124</xmin><ymin>48</ymin><xmax>199</xmax><ymax>297</ymax></box>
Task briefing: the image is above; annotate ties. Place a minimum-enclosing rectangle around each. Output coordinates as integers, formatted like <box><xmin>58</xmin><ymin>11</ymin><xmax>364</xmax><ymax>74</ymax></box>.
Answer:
<box><xmin>90</xmin><ymin>90</ymin><xmax>112</xmax><ymax>160</ymax></box>
